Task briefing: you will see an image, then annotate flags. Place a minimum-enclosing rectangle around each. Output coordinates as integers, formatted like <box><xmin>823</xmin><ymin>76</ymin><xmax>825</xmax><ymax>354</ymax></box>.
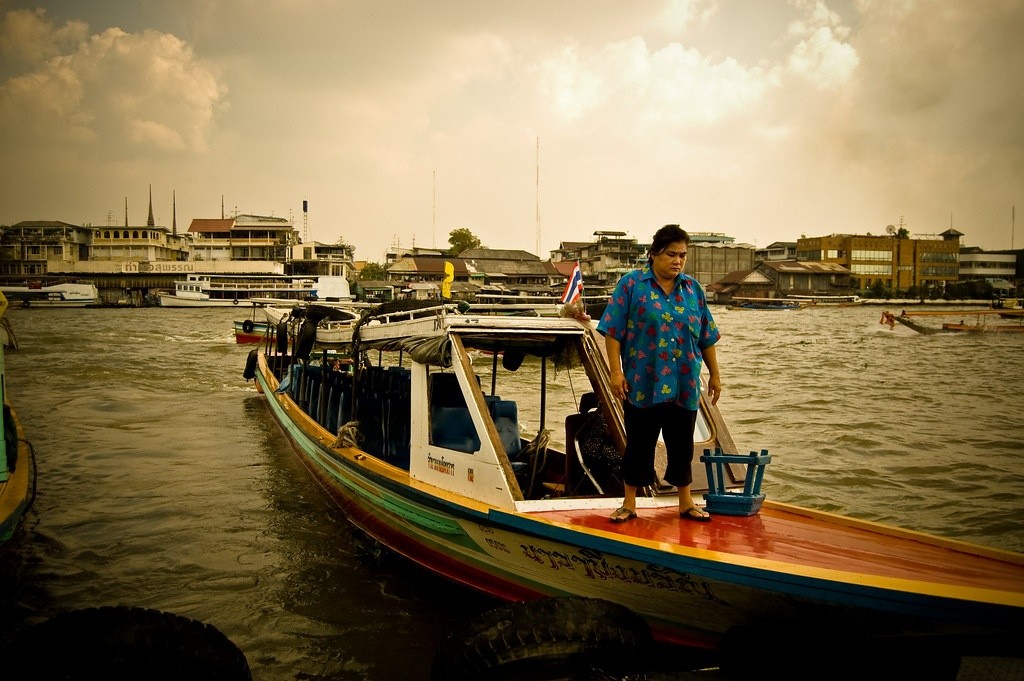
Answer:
<box><xmin>443</xmin><ymin>262</ymin><xmax>454</xmax><ymax>300</ymax></box>
<box><xmin>562</xmin><ymin>260</ymin><xmax>583</xmax><ymax>306</ymax></box>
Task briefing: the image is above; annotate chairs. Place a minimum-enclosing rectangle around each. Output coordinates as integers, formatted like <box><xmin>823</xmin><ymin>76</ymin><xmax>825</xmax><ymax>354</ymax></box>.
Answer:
<box><xmin>294</xmin><ymin>366</ymin><xmax>411</xmax><ymax>470</ymax></box>
<box><xmin>564</xmin><ymin>393</ymin><xmax>598</xmax><ymax>497</ymax></box>
<box><xmin>491</xmin><ymin>400</ymin><xmax>522</xmax><ymax>457</ymax></box>
<box><xmin>428</xmin><ymin>372</ymin><xmax>480</xmax><ymax>454</ymax></box>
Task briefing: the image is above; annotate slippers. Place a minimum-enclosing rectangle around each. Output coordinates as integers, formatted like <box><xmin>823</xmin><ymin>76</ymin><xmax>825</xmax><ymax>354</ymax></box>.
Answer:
<box><xmin>609</xmin><ymin>507</ymin><xmax>638</xmax><ymax>523</ymax></box>
<box><xmin>679</xmin><ymin>505</ymin><xmax>711</xmax><ymax>522</ymax></box>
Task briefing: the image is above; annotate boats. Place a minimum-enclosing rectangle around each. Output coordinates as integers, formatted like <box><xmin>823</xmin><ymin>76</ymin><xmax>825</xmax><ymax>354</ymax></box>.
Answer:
<box><xmin>243</xmin><ymin>298</ymin><xmax>1024</xmax><ymax>659</ymax></box>
<box><xmin>1</xmin><ymin>283</ymin><xmax>104</xmax><ymax>308</ymax></box>
<box><xmin>232</xmin><ymin>320</ymin><xmax>315</xmax><ymax>345</ymax></box>
<box><xmin>361</xmin><ymin>282</ymin><xmax>612</xmax><ymax>320</ymax></box>
<box><xmin>157</xmin><ymin>273</ymin><xmax>357</xmax><ymax>307</ymax></box>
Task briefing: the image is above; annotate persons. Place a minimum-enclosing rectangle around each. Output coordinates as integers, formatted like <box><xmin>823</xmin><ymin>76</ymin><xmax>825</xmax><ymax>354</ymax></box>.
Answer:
<box><xmin>308</xmin><ymin>355</ymin><xmax>354</xmax><ymax>372</ymax></box>
<box><xmin>594</xmin><ymin>224</ymin><xmax>722</xmax><ymax>522</ymax></box>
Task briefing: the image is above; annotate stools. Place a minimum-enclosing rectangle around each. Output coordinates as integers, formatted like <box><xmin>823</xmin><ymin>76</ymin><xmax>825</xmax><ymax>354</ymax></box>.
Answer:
<box><xmin>700</xmin><ymin>446</ymin><xmax>771</xmax><ymax>517</ymax></box>
